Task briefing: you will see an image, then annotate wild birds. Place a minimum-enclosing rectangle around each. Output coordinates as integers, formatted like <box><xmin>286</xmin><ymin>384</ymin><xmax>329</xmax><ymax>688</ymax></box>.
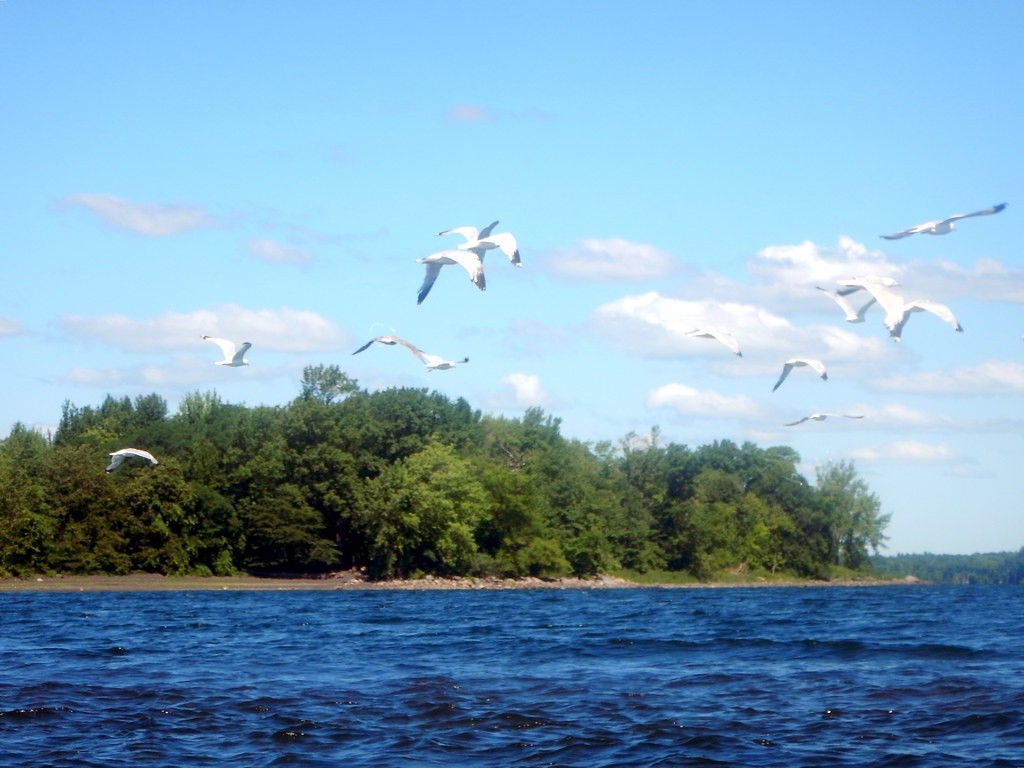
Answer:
<box><xmin>817</xmin><ymin>280</ymin><xmax>966</xmax><ymax>342</ymax></box>
<box><xmin>415</xmin><ymin>249</ymin><xmax>488</xmax><ymax>304</ymax></box>
<box><xmin>432</xmin><ymin>221</ymin><xmax>522</xmax><ymax>270</ymax></box>
<box><xmin>352</xmin><ymin>335</ymin><xmax>470</xmax><ymax>373</ymax></box>
<box><xmin>878</xmin><ymin>201</ymin><xmax>1010</xmax><ymax>241</ymax></box>
<box><xmin>104</xmin><ymin>447</ymin><xmax>160</xmax><ymax>475</ymax></box>
<box><xmin>783</xmin><ymin>411</ymin><xmax>866</xmax><ymax>432</ymax></box>
<box><xmin>771</xmin><ymin>357</ymin><xmax>831</xmax><ymax>391</ymax></box>
<box><xmin>201</xmin><ymin>334</ymin><xmax>251</xmax><ymax>367</ymax></box>
<box><xmin>688</xmin><ymin>326</ymin><xmax>743</xmax><ymax>358</ymax></box>
